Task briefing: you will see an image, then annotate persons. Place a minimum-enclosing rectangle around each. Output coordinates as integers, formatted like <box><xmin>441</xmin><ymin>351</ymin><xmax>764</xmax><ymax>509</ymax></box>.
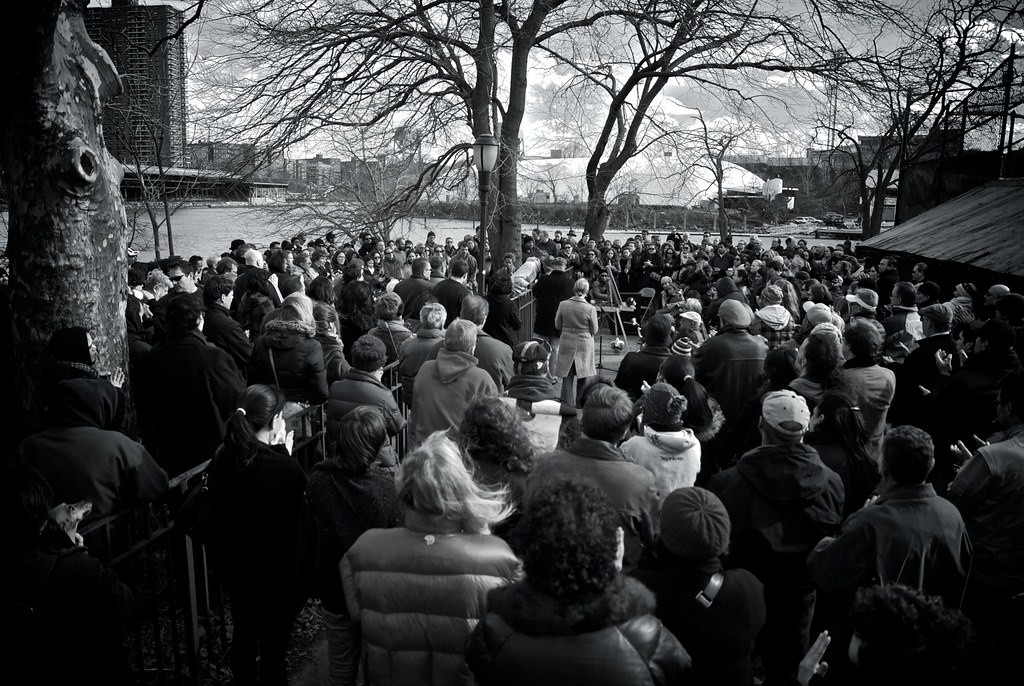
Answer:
<box><xmin>0</xmin><ymin>226</ymin><xmax>1024</xmax><ymax>686</ymax></box>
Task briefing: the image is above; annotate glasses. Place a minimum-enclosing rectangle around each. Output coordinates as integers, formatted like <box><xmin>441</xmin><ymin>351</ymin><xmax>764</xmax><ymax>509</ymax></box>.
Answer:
<box><xmin>648</xmin><ymin>248</ymin><xmax>655</xmax><ymax>251</ymax></box>
<box><xmin>317</xmin><ymin>244</ymin><xmax>326</xmax><ymax>247</ymax></box>
<box><xmin>169</xmin><ymin>275</ymin><xmax>183</xmax><ymax>282</ymax></box>
<box><xmin>644</xmin><ymin>243</ymin><xmax>650</xmax><ymax>247</ymax></box>
<box><xmin>600</xmin><ymin>274</ymin><xmax>608</xmax><ymax>277</ymax></box>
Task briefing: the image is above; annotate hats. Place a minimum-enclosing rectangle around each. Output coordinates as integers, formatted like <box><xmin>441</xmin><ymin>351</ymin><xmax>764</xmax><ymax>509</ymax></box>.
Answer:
<box><xmin>718</xmin><ymin>299</ymin><xmax>752</xmax><ymax>327</ymax></box>
<box><xmin>669</xmin><ymin>337</ymin><xmax>692</xmax><ymax>357</ymax></box>
<box><xmin>760</xmin><ymin>389</ymin><xmax>812</xmax><ymax>436</ymax></box>
<box><xmin>679</xmin><ymin>311</ymin><xmax>703</xmax><ymax>323</ymax></box>
<box><xmin>511</xmin><ymin>336</ymin><xmax>552</xmax><ymax>363</ymax></box>
<box><xmin>566</xmin><ymin>230</ymin><xmax>577</xmax><ymax>237</ymax></box>
<box><xmin>229</xmin><ymin>240</ymin><xmax>246</xmax><ymax>250</ymax></box>
<box><xmin>761</xmin><ymin>284</ymin><xmax>783</xmax><ymax>304</ymax></box>
<box><xmin>845</xmin><ymin>289</ymin><xmax>879</xmax><ymax>312</ymax></box>
<box><xmin>660</xmin><ymin>485</ymin><xmax>731</xmax><ymax>558</ymax></box>
<box><xmin>803</xmin><ymin>301</ymin><xmax>835</xmax><ymax>326</ymax></box>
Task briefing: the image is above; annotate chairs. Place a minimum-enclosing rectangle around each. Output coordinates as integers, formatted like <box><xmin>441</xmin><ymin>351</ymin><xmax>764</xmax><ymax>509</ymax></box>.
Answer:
<box><xmin>622</xmin><ymin>288</ymin><xmax>656</xmax><ymax>331</ymax></box>
